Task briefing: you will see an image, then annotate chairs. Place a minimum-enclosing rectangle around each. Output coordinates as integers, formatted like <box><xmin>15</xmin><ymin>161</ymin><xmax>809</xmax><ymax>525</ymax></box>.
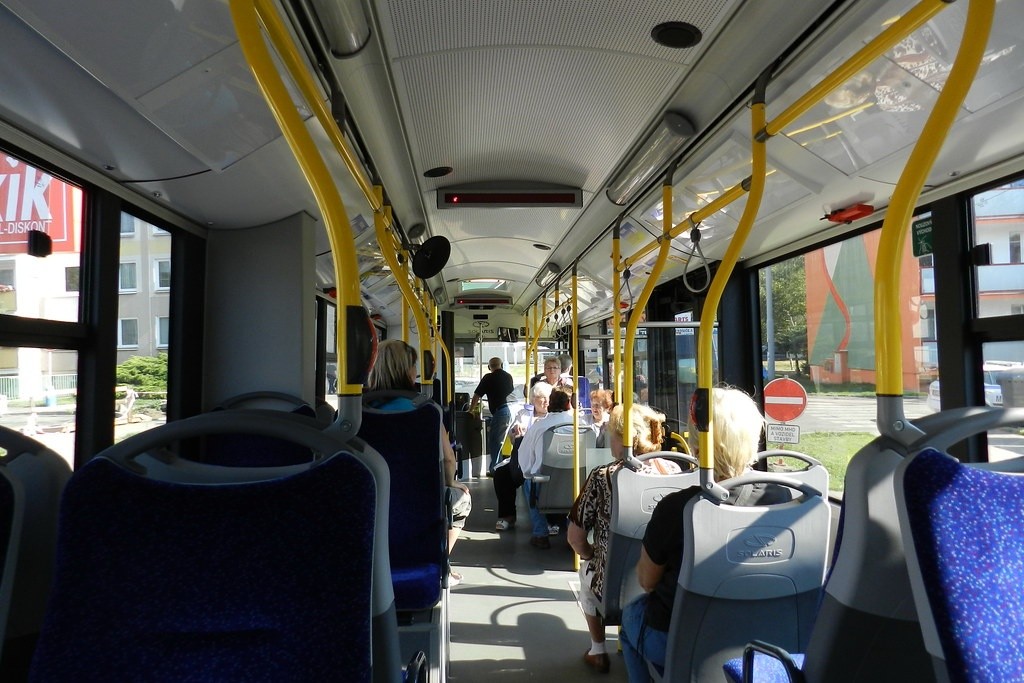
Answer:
<box><xmin>529</xmin><ymin>423</ymin><xmax>596</xmax><ymax>515</ymax></box>
<box><xmin>586</xmin><ymin>450</ymin><xmax>700</xmax><ymax>627</ymax></box>
<box><xmin>128</xmin><ymin>406</ymin><xmax>428</xmax><ymax>683</ymax></box>
<box><xmin>748</xmin><ymin>448</ymin><xmax>828</xmax><ymax>498</ymax></box>
<box><xmin>359</xmin><ymin>388</ymin><xmax>452</xmax><ymax>613</ymax></box>
<box><xmin>899</xmin><ymin>407</ymin><xmax>1024</xmax><ymax>683</ymax></box>
<box><xmin>0</xmin><ymin>424</ymin><xmax>73</xmax><ymax>683</ymax></box>
<box><xmin>216</xmin><ymin>391</ymin><xmax>335</xmax><ymax>428</ymax></box>
<box><xmin>723</xmin><ymin>407</ymin><xmax>1008</xmax><ymax>683</ymax></box>
<box><xmin>27</xmin><ymin>413</ymin><xmax>379</xmax><ymax>683</ymax></box>
<box><xmin>634</xmin><ymin>470</ymin><xmax>833</xmax><ymax>683</ymax></box>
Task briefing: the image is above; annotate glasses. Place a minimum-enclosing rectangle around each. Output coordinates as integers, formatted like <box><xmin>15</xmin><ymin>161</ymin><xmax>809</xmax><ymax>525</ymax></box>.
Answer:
<box><xmin>544</xmin><ymin>366</ymin><xmax>559</xmax><ymax>372</ymax></box>
<box><xmin>396</xmin><ymin>339</ymin><xmax>408</xmax><ymax>351</ymax></box>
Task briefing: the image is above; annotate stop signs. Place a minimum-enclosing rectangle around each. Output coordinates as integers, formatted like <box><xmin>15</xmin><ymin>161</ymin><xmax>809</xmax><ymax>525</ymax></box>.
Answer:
<box><xmin>764</xmin><ymin>374</ymin><xmax>808</xmax><ymax>423</ymax></box>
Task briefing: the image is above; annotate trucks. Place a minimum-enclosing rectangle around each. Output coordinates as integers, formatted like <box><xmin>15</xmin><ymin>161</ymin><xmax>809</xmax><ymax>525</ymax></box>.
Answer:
<box><xmin>598</xmin><ymin>321</ymin><xmax>719</xmax><ymax>423</ymax></box>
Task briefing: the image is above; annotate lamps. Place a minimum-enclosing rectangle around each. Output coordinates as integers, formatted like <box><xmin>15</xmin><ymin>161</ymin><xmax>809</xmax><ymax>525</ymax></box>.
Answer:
<box><xmin>605</xmin><ymin>110</ymin><xmax>696</xmax><ymax>206</ymax></box>
<box><xmin>433</xmin><ymin>287</ymin><xmax>446</xmax><ymax>305</ymax></box>
<box><xmin>535</xmin><ymin>262</ymin><xmax>560</xmax><ymax>289</ymax></box>
<box><xmin>311</xmin><ymin>0</ymin><xmax>373</xmax><ymax>62</ymax></box>
<box><xmin>407</xmin><ymin>223</ymin><xmax>428</xmax><ymax>247</ymax></box>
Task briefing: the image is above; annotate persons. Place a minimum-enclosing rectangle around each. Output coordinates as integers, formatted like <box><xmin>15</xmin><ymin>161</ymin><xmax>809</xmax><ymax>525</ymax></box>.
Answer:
<box><xmin>568</xmin><ymin>402</ymin><xmax>682</xmax><ymax>674</ymax></box>
<box><xmin>518</xmin><ymin>384</ymin><xmax>601</xmax><ymax>546</ymax></box>
<box><xmin>363</xmin><ymin>338</ymin><xmax>471</xmax><ymax>588</ymax></box>
<box><xmin>470</xmin><ymin>357</ymin><xmax>514</xmax><ymax>478</ymax></box>
<box><xmin>493</xmin><ymin>381</ymin><xmax>554</xmax><ymax>531</ymax></box>
<box><xmin>618</xmin><ymin>372</ymin><xmax>804</xmax><ymax>683</ymax></box>
<box><xmin>523</xmin><ymin>355</ymin><xmax>585</xmax><ymax>417</ymax></box>
<box><xmin>581</xmin><ymin>389</ymin><xmax>613</xmax><ymax>439</ymax></box>
<box><xmin>498</xmin><ymin>328</ymin><xmax>510</xmax><ymax>341</ymax></box>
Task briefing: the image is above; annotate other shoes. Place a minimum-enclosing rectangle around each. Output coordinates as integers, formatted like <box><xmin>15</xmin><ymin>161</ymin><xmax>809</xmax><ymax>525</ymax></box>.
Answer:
<box><xmin>496</xmin><ymin>520</ymin><xmax>515</xmax><ymax>530</ymax></box>
<box><xmin>549</xmin><ymin>525</ymin><xmax>560</xmax><ymax>534</ymax></box>
<box><xmin>584</xmin><ymin>648</ymin><xmax>610</xmax><ymax>673</ymax></box>
<box><xmin>528</xmin><ymin>535</ymin><xmax>551</xmax><ymax>549</ymax></box>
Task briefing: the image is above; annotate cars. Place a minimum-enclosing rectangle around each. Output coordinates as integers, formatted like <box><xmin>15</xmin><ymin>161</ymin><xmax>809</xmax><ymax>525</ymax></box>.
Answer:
<box><xmin>925</xmin><ymin>359</ymin><xmax>1024</xmax><ymax>413</ymax></box>
